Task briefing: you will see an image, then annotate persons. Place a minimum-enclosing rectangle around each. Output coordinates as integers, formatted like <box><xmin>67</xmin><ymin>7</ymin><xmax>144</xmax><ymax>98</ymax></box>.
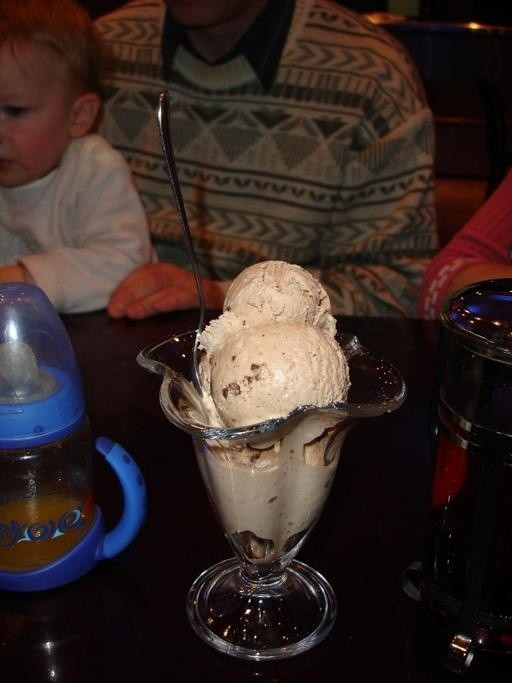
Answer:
<box><xmin>91</xmin><ymin>0</ymin><xmax>441</xmax><ymax>323</ymax></box>
<box><xmin>415</xmin><ymin>164</ymin><xmax>511</xmax><ymax>326</ymax></box>
<box><xmin>1</xmin><ymin>0</ymin><xmax>158</xmax><ymax>318</ymax></box>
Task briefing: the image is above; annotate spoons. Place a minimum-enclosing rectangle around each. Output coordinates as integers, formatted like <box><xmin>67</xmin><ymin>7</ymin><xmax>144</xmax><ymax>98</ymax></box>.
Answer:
<box><xmin>155</xmin><ymin>88</ymin><xmax>207</xmax><ymax>397</ymax></box>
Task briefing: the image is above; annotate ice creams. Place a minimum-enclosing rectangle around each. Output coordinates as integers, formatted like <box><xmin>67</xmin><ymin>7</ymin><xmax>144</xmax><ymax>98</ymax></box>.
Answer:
<box><xmin>182</xmin><ymin>259</ymin><xmax>352</xmax><ymax>580</ymax></box>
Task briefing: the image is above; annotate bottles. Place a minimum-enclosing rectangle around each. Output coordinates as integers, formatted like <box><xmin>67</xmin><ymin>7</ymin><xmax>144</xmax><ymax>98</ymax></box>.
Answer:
<box><xmin>398</xmin><ymin>277</ymin><xmax>512</xmax><ymax>683</ymax></box>
<box><xmin>0</xmin><ymin>278</ymin><xmax>147</xmax><ymax>595</ymax></box>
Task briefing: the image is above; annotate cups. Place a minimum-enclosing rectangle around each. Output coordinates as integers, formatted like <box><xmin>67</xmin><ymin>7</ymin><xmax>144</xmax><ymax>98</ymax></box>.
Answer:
<box><xmin>135</xmin><ymin>320</ymin><xmax>407</xmax><ymax>663</ymax></box>
